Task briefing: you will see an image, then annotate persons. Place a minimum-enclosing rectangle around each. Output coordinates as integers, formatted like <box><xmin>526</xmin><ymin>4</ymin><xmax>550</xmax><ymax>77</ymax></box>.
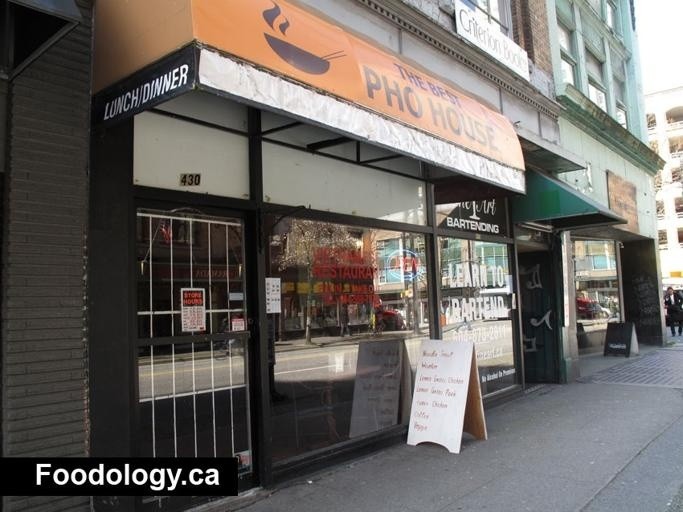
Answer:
<box><xmin>664</xmin><ymin>287</ymin><xmax>683</xmax><ymax>336</ymax></box>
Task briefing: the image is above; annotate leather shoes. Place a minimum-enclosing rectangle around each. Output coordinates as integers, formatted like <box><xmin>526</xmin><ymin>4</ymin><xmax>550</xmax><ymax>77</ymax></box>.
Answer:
<box><xmin>673</xmin><ymin>330</ymin><xmax>681</xmax><ymax>336</ymax></box>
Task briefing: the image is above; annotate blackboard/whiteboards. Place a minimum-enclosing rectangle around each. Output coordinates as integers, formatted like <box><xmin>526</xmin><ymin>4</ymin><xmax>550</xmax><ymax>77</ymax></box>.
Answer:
<box><xmin>349</xmin><ymin>338</ymin><xmax>404</xmax><ymax>439</ymax></box>
<box><xmin>604</xmin><ymin>323</ymin><xmax>633</xmax><ymax>354</ymax></box>
<box><xmin>406</xmin><ymin>338</ymin><xmax>474</xmax><ymax>454</ymax></box>
